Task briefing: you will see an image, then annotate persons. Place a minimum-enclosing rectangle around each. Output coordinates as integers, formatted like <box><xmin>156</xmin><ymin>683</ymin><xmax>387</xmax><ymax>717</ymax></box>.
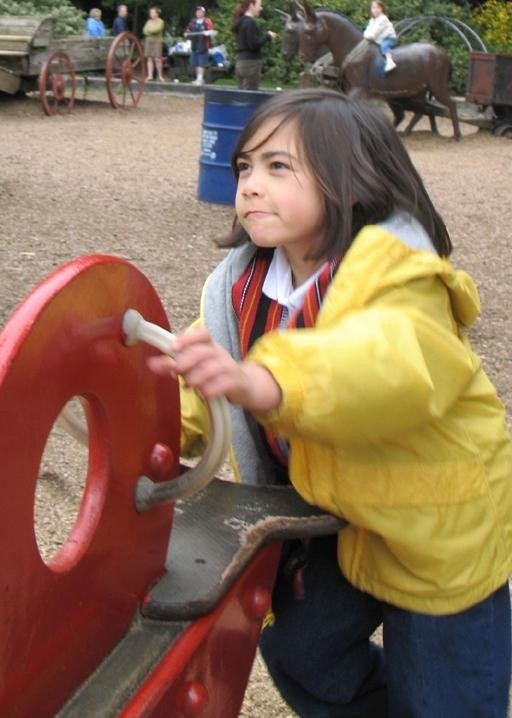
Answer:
<box><xmin>230</xmin><ymin>0</ymin><xmax>278</xmax><ymax>90</ymax></box>
<box><xmin>85</xmin><ymin>7</ymin><xmax>103</xmax><ymax>37</ymax></box>
<box><xmin>111</xmin><ymin>4</ymin><xmax>131</xmax><ymax>35</ymax></box>
<box><xmin>182</xmin><ymin>5</ymin><xmax>216</xmax><ymax>84</ymax></box>
<box><xmin>147</xmin><ymin>86</ymin><xmax>512</xmax><ymax>717</ymax></box>
<box><xmin>141</xmin><ymin>7</ymin><xmax>164</xmax><ymax>82</ymax></box>
<box><xmin>361</xmin><ymin>0</ymin><xmax>399</xmax><ymax>73</ymax></box>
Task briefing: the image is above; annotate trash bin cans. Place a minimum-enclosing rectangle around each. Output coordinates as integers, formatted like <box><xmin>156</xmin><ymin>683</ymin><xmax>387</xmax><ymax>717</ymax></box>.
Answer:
<box><xmin>196</xmin><ymin>87</ymin><xmax>284</xmax><ymax>206</ymax></box>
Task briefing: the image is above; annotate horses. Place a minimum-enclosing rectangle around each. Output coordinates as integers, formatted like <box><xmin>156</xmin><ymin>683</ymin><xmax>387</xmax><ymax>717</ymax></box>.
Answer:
<box><xmin>279</xmin><ymin>0</ymin><xmax>463</xmax><ymax>144</ymax></box>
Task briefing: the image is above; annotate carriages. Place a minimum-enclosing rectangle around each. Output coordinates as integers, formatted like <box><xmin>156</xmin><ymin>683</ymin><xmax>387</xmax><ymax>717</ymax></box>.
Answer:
<box><xmin>277</xmin><ymin>0</ymin><xmax>512</xmax><ymax>144</ymax></box>
